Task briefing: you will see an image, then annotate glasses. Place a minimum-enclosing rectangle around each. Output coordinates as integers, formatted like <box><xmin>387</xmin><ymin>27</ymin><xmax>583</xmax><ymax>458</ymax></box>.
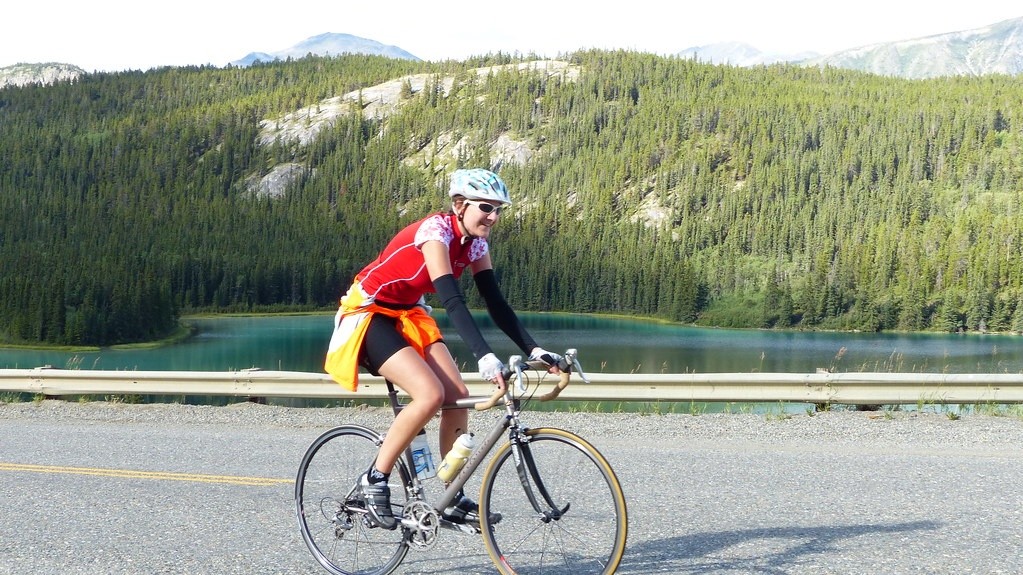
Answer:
<box><xmin>464</xmin><ymin>199</ymin><xmax>504</xmax><ymax>215</ymax></box>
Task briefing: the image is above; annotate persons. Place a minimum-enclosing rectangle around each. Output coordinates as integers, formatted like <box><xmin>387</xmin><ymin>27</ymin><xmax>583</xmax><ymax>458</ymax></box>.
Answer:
<box><xmin>324</xmin><ymin>168</ymin><xmax>571</xmax><ymax>530</ymax></box>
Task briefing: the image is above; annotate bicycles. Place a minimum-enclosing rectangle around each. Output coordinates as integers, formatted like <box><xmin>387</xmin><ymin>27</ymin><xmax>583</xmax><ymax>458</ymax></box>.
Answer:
<box><xmin>296</xmin><ymin>347</ymin><xmax>628</xmax><ymax>575</ymax></box>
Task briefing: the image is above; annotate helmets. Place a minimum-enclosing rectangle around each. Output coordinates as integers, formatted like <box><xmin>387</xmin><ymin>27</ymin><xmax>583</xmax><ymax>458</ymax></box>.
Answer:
<box><xmin>448</xmin><ymin>169</ymin><xmax>512</xmax><ymax>205</ymax></box>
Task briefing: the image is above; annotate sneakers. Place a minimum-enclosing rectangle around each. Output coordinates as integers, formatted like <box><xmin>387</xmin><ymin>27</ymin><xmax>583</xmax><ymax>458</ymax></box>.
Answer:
<box><xmin>442</xmin><ymin>496</ymin><xmax>502</xmax><ymax>524</ymax></box>
<box><xmin>357</xmin><ymin>470</ymin><xmax>398</xmax><ymax>531</ymax></box>
